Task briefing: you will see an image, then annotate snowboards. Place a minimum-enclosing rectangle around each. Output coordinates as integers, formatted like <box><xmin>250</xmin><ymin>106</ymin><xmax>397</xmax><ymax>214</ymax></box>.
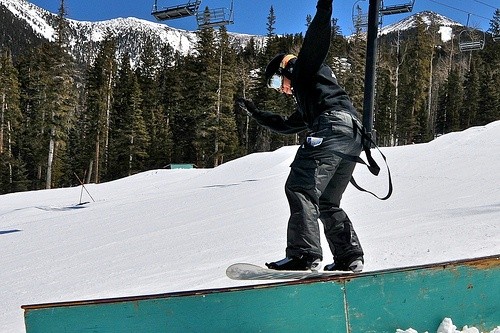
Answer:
<box><xmin>226</xmin><ymin>262</ymin><xmax>361</xmax><ymax>280</ymax></box>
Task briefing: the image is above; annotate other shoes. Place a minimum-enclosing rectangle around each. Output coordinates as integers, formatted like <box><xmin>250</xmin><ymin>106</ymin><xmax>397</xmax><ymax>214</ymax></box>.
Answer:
<box><xmin>267</xmin><ymin>256</ymin><xmax>319</xmax><ymax>271</ymax></box>
<box><xmin>325</xmin><ymin>260</ymin><xmax>364</xmax><ymax>272</ymax></box>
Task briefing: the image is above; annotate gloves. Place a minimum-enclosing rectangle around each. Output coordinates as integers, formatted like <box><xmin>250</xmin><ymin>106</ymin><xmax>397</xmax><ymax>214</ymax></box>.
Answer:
<box><xmin>237</xmin><ymin>97</ymin><xmax>256</xmax><ymax>114</ymax></box>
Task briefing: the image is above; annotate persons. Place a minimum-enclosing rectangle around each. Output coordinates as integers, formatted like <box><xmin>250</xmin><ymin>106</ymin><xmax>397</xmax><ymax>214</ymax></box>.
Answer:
<box><xmin>236</xmin><ymin>0</ymin><xmax>367</xmax><ymax>271</ymax></box>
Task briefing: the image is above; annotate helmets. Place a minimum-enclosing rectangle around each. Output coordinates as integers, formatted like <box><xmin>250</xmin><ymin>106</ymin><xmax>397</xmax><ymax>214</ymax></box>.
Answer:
<box><xmin>263</xmin><ymin>54</ymin><xmax>296</xmax><ymax>89</ymax></box>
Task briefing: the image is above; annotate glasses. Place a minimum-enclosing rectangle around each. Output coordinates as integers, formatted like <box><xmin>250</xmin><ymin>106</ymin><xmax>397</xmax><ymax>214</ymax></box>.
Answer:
<box><xmin>267</xmin><ymin>68</ymin><xmax>285</xmax><ymax>92</ymax></box>
